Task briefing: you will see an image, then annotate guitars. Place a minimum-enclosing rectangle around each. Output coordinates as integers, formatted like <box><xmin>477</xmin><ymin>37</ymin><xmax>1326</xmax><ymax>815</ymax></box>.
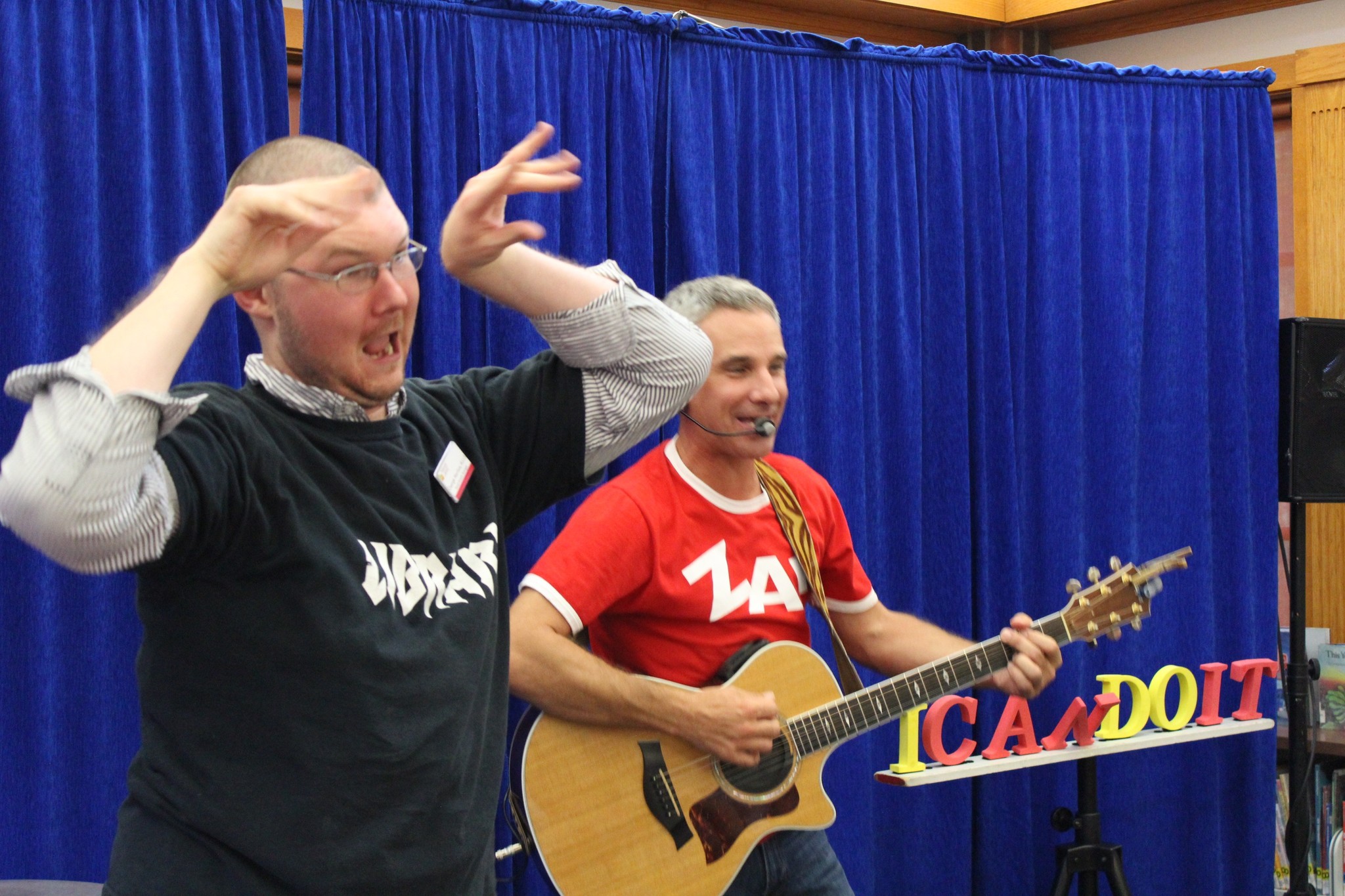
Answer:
<box><xmin>507</xmin><ymin>542</ymin><xmax>1197</xmax><ymax>895</ymax></box>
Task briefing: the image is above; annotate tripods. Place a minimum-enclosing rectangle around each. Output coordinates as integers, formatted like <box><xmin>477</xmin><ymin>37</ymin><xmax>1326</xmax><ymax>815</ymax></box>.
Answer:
<box><xmin>1039</xmin><ymin>757</ymin><xmax>1135</xmax><ymax>896</ymax></box>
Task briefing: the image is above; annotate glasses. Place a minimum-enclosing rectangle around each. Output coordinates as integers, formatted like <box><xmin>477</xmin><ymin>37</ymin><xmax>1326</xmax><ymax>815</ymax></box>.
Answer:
<box><xmin>284</xmin><ymin>238</ymin><xmax>427</xmax><ymax>294</ymax></box>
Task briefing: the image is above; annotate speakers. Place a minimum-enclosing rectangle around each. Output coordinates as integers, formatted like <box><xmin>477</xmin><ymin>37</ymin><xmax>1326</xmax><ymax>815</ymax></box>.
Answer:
<box><xmin>1278</xmin><ymin>316</ymin><xmax>1345</xmax><ymax>505</ymax></box>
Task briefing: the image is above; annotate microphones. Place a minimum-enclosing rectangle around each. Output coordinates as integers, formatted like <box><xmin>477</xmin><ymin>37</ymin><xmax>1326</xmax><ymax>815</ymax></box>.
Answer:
<box><xmin>677</xmin><ymin>410</ymin><xmax>778</xmax><ymax>439</ymax></box>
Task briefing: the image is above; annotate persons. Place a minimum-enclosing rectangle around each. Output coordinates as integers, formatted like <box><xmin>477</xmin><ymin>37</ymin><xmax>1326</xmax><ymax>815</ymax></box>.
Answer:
<box><xmin>507</xmin><ymin>279</ymin><xmax>1062</xmax><ymax>896</ymax></box>
<box><xmin>0</xmin><ymin>119</ymin><xmax>712</xmax><ymax>896</ymax></box>
